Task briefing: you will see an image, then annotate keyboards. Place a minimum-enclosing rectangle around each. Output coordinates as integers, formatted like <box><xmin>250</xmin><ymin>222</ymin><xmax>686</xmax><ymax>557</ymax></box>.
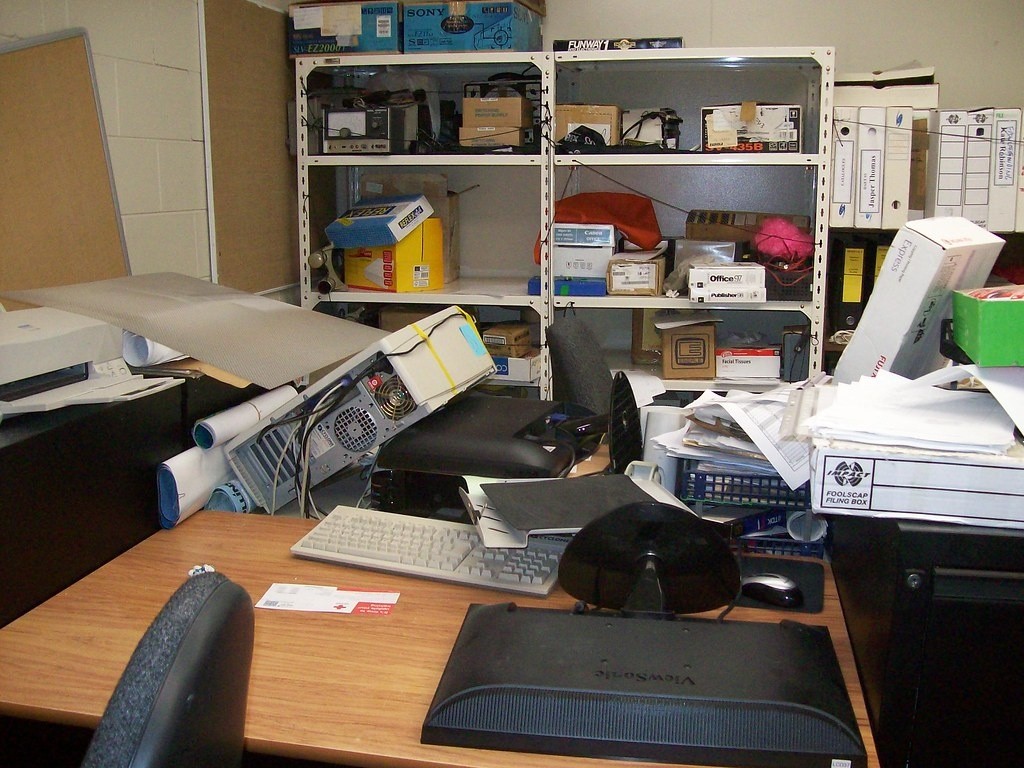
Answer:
<box><xmin>222</xmin><ymin>306</ymin><xmax>498</xmax><ymax>513</ymax></box>
<box><xmin>290</xmin><ymin>505</ymin><xmax>574</xmax><ymax>597</ymax></box>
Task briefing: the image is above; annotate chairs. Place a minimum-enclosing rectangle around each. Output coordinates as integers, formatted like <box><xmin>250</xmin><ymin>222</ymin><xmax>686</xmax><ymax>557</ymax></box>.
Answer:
<box><xmin>79</xmin><ymin>569</ymin><xmax>255</xmax><ymax>768</ymax></box>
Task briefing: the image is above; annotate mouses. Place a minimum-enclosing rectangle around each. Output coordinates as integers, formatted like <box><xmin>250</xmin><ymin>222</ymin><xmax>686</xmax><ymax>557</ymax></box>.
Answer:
<box><xmin>740</xmin><ymin>572</ymin><xmax>805</xmax><ymax>608</ymax></box>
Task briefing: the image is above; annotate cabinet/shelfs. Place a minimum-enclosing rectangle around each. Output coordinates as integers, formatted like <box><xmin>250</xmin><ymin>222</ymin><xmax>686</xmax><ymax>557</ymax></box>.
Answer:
<box><xmin>145</xmin><ymin>373</ymin><xmax>267</xmax><ymax>450</ymax></box>
<box><xmin>879</xmin><ymin>521</ymin><xmax>1024</xmax><ymax>768</ymax></box>
<box><xmin>291</xmin><ymin>50</ymin><xmax>837</xmax><ymax>395</ymax></box>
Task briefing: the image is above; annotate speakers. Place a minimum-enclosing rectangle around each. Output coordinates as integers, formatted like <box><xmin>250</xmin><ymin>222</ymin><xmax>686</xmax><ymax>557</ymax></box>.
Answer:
<box><xmin>547</xmin><ymin>318</ymin><xmax>614</xmax><ymax>414</ymax></box>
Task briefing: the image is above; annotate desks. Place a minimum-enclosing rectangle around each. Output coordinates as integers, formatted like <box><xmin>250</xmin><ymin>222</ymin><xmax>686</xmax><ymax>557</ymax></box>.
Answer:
<box><xmin>1</xmin><ymin>505</ymin><xmax>876</xmax><ymax>768</ymax></box>
<box><xmin>1</xmin><ymin>379</ymin><xmax>185</xmax><ymax>629</ymax></box>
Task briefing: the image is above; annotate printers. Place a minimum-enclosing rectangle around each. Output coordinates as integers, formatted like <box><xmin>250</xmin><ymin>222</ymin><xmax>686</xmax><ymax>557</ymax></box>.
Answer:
<box><xmin>0</xmin><ymin>307</ymin><xmax>185</xmax><ymax>420</ymax></box>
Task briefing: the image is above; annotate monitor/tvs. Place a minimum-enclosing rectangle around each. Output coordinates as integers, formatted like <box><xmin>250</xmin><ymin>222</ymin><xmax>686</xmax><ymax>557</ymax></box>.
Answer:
<box><xmin>377</xmin><ymin>371</ymin><xmax>644</xmax><ymax>483</ymax></box>
<box><xmin>420</xmin><ymin>500</ymin><xmax>868</xmax><ymax>768</ymax></box>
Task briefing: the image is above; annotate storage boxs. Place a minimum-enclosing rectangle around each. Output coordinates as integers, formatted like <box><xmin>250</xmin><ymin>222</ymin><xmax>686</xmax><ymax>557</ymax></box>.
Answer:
<box><xmin>319</xmin><ymin>65</ymin><xmax>1024</xmax><ymax>528</ymax></box>
<box><xmin>676</xmin><ymin>395</ymin><xmax>811</xmax><ymax>507</ymax></box>
<box><xmin>552</xmin><ymin>35</ymin><xmax>686</xmax><ymax>48</ymax></box>
<box><xmin>401</xmin><ymin>0</ymin><xmax>549</xmax><ymax>54</ymax></box>
<box><xmin>287</xmin><ymin>1</ymin><xmax>403</xmax><ymax>55</ymax></box>
